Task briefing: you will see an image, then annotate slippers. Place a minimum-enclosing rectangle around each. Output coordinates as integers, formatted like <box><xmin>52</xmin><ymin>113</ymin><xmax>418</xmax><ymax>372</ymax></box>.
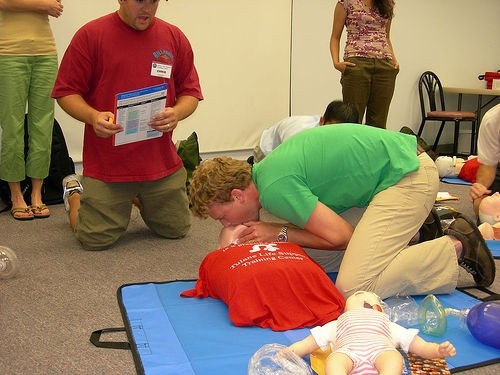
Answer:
<box><xmin>11</xmin><ymin>206</ymin><xmax>34</xmax><ymax>220</ymax></box>
<box><xmin>31</xmin><ymin>204</ymin><xmax>50</xmax><ymax>216</ymax></box>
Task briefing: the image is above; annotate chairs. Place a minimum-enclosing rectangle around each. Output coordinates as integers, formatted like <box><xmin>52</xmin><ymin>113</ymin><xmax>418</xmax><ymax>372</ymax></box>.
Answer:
<box><xmin>417</xmin><ymin>71</ymin><xmax>476</xmax><ymax>158</ymax></box>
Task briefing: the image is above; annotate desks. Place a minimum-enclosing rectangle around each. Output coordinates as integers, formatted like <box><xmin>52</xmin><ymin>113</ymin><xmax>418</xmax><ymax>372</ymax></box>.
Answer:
<box><xmin>443</xmin><ymin>86</ymin><xmax>500</xmax><ymax>157</ymax></box>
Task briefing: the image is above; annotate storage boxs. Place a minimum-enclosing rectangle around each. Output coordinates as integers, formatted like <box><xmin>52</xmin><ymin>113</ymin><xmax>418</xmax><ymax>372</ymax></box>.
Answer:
<box><xmin>457</xmin><ymin>130</ymin><xmax>478</xmax><ymax>155</ymax></box>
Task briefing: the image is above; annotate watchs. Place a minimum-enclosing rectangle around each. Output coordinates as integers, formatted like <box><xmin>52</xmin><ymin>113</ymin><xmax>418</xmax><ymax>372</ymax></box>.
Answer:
<box><xmin>277</xmin><ymin>226</ymin><xmax>288</xmax><ymax>241</ymax></box>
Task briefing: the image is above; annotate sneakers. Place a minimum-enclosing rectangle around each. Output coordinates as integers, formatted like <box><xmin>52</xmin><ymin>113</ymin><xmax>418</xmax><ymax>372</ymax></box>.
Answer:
<box><xmin>444</xmin><ymin>215</ymin><xmax>496</xmax><ymax>286</ymax></box>
<box><xmin>62</xmin><ymin>174</ymin><xmax>83</xmax><ymax>223</ymax></box>
<box><xmin>416</xmin><ymin>207</ymin><xmax>444</xmax><ymax>245</ymax></box>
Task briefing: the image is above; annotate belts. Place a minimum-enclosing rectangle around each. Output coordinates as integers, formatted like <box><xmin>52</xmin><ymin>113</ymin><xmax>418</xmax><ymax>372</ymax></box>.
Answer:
<box><xmin>417</xmin><ymin>144</ymin><xmax>425</xmax><ymax>157</ymax></box>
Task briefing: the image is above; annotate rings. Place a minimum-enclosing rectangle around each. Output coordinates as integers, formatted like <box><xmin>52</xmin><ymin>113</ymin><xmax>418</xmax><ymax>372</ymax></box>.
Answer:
<box><xmin>168</xmin><ymin>123</ymin><xmax>170</xmax><ymax>128</ymax></box>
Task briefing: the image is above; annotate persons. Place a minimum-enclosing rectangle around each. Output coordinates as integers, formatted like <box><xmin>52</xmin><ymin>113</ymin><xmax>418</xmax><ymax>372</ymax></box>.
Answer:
<box><xmin>278</xmin><ymin>290</ymin><xmax>456</xmax><ymax>375</ymax></box>
<box><xmin>51</xmin><ymin>0</ymin><xmax>203</xmax><ymax>251</ymax></box>
<box><xmin>469</xmin><ymin>103</ymin><xmax>500</xmax><ymax>201</ymax></box>
<box><xmin>189</xmin><ymin>123</ymin><xmax>496</xmax><ymax>301</ymax></box>
<box><xmin>330</xmin><ymin>0</ymin><xmax>399</xmax><ymax>128</ymax></box>
<box><xmin>181</xmin><ymin>224</ymin><xmax>345</xmax><ymax>331</ymax></box>
<box><xmin>246</xmin><ymin>100</ymin><xmax>358</xmax><ymax>166</ymax></box>
<box><xmin>0</xmin><ymin>0</ymin><xmax>63</xmax><ymax>220</ymax></box>
<box><xmin>435</xmin><ymin>154</ymin><xmax>481</xmax><ymax>183</ymax></box>
<box><xmin>479</xmin><ymin>191</ymin><xmax>500</xmax><ymax>225</ymax></box>
